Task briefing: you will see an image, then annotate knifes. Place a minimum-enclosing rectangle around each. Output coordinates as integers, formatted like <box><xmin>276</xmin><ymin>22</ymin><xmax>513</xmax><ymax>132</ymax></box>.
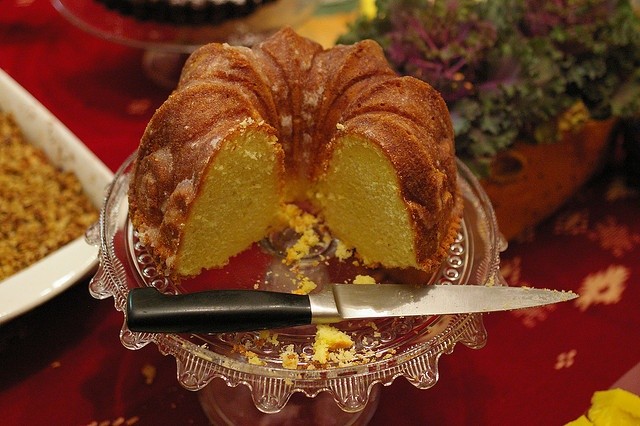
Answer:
<box><xmin>127</xmin><ymin>283</ymin><xmax>580</xmax><ymax>334</ymax></box>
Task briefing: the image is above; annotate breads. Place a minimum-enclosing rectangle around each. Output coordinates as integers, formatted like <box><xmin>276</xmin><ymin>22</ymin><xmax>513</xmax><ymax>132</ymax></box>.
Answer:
<box><xmin>128</xmin><ymin>26</ymin><xmax>464</xmax><ymax>280</ymax></box>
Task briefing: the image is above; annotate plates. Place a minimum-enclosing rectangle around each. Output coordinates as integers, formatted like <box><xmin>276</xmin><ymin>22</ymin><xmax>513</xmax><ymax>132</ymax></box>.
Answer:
<box><xmin>0</xmin><ymin>67</ymin><xmax>131</xmax><ymax>324</ymax></box>
